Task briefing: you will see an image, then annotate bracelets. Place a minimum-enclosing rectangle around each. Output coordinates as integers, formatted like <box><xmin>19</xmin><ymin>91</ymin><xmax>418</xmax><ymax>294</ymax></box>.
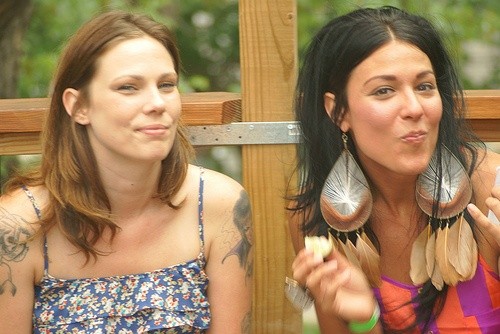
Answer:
<box><xmin>347</xmin><ymin>304</ymin><xmax>380</xmax><ymax>334</ymax></box>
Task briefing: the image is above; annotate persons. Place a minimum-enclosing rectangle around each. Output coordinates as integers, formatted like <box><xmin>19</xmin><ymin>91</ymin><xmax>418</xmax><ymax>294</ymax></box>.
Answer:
<box><xmin>282</xmin><ymin>6</ymin><xmax>500</xmax><ymax>334</ymax></box>
<box><xmin>0</xmin><ymin>9</ymin><xmax>255</xmax><ymax>334</ymax></box>
